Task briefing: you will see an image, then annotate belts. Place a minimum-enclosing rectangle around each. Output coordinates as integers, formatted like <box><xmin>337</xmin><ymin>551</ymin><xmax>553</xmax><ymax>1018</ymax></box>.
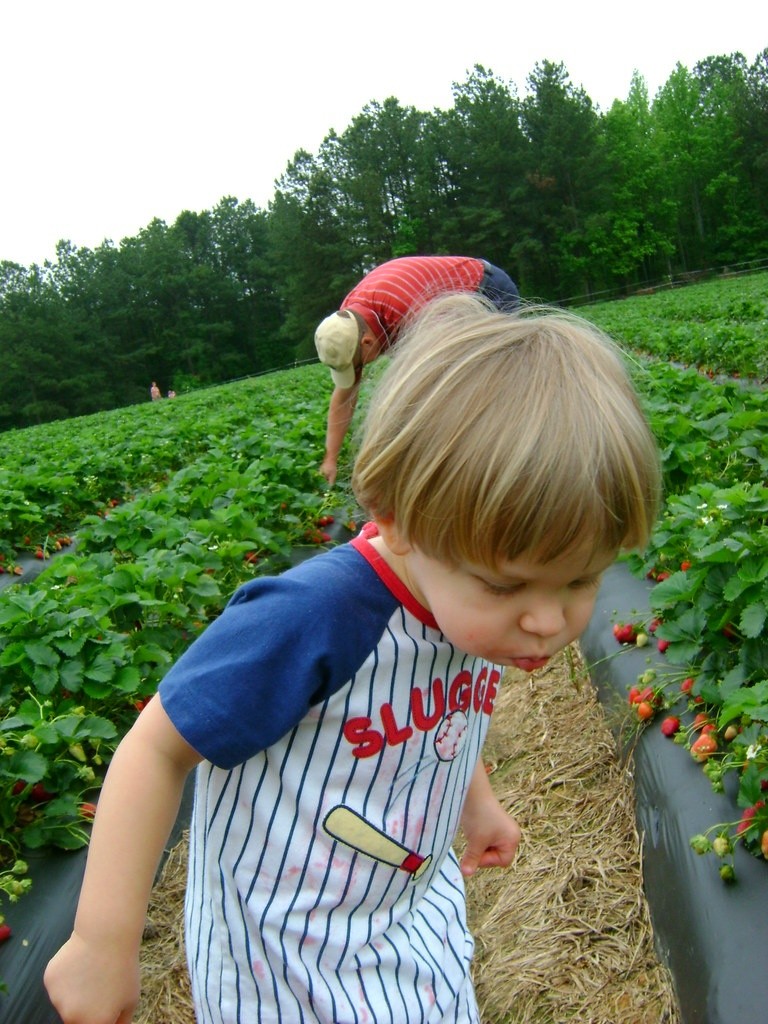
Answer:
<box><xmin>478</xmin><ymin>258</ymin><xmax>491</xmax><ymax>294</ymax></box>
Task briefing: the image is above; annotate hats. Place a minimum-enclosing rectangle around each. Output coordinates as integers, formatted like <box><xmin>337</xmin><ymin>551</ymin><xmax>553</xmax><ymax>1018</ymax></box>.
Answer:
<box><xmin>314</xmin><ymin>309</ymin><xmax>361</xmax><ymax>390</ymax></box>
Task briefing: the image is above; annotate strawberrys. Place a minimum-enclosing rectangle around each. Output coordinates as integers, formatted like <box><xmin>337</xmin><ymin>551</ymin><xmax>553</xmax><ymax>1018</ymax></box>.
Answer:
<box><xmin>1</xmin><ymin>496</ymin><xmax>356</xmax><ymax>939</ymax></box>
<box><xmin>613</xmin><ymin>557</ymin><xmax>768</xmax><ymax>881</ymax></box>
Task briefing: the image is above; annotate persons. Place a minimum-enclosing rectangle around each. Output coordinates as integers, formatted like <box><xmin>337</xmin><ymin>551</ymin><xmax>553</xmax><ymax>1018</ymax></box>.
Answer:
<box><xmin>152</xmin><ymin>381</ymin><xmax>175</xmax><ymax>398</ymax></box>
<box><xmin>313</xmin><ymin>257</ymin><xmax>520</xmax><ymax>486</ymax></box>
<box><xmin>42</xmin><ymin>284</ymin><xmax>659</xmax><ymax>1024</ymax></box>
